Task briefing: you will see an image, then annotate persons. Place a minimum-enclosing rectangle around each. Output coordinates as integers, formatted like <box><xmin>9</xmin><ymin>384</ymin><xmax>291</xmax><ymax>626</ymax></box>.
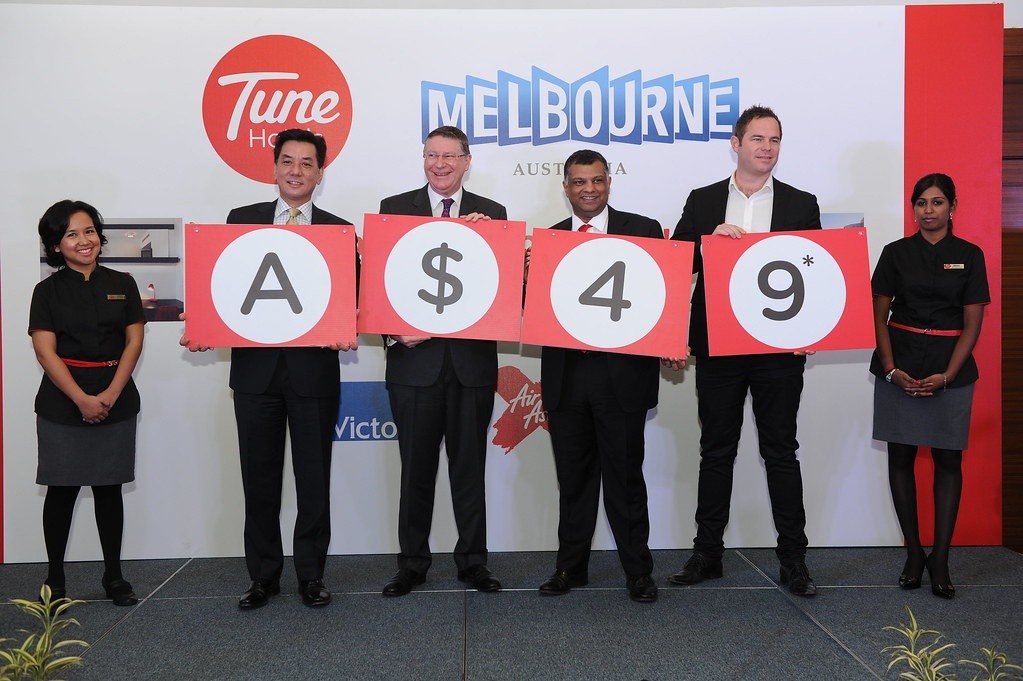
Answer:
<box><xmin>28</xmin><ymin>199</ymin><xmax>147</xmax><ymax>617</ymax></box>
<box><xmin>668</xmin><ymin>104</ymin><xmax>823</xmax><ymax>596</ymax></box>
<box><xmin>181</xmin><ymin>129</ymin><xmax>361</xmax><ymax>606</ymax></box>
<box><xmin>523</xmin><ymin>149</ymin><xmax>691</xmax><ymax>600</ymax></box>
<box><xmin>869</xmin><ymin>172</ymin><xmax>991</xmax><ymax>599</ymax></box>
<box><xmin>377</xmin><ymin>127</ymin><xmax>508</xmax><ymax>598</ymax></box>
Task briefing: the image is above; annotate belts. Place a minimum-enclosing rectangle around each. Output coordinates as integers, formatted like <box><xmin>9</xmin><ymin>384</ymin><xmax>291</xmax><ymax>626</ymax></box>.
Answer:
<box><xmin>61</xmin><ymin>358</ymin><xmax>119</xmax><ymax>368</ymax></box>
<box><xmin>888</xmin><ymin>321</ymin><xmax>962</xmax><ymax>336</ymax></box>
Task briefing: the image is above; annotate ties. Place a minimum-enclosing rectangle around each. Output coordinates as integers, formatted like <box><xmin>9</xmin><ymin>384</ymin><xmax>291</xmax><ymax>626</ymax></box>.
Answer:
<box><xmin>286</xmin><ymin>208</ymin><xmax>302</xmax><ymax>224</ymax></box>
<box><xmin>578</xmin><ymin>224</ymin><xmax>593</xmax><ymax>232</ymax></box>
<box><xmin>440</xmin><ymin>198</ymin><xmax>455</xmax><ymax>217</ymax></box>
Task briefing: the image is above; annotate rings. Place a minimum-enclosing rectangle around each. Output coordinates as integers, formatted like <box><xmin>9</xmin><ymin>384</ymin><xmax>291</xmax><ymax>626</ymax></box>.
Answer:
<box><xmin>913</xmin><ymin>391</ymin><xmax>917</xmax><ymax>398</ymax></box>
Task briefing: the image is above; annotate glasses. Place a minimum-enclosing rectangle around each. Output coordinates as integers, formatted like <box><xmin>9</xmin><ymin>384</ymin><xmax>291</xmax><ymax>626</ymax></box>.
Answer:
<box><xmin>423</xmin><ymin>152</ymin><xmax>466</xmax><ymax>161</ymax></box>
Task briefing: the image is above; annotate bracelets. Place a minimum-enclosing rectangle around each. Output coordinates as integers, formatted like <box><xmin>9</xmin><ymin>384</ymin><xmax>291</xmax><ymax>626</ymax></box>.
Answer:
<box><xmin>942</xmin><ymin>373</ymin><xmax>946</xmax><ymax>393</ymax></box>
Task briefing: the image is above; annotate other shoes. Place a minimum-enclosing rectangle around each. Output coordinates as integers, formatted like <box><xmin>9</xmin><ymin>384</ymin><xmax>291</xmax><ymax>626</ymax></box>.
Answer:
<box><xmin>102</xmin><ymin>575</ymin><xmax>138</xmax><ymax>606</ymax></box>
<box><xmin>40</xmin><ymin>578</ymin><xmax>67</xmax><ymax>615</ymax></box>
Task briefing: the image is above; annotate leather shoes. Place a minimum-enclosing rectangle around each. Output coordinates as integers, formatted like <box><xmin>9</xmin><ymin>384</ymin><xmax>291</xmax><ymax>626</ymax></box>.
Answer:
<box><xmin>298</xmin><ymin>579</ymin><xmax>331</xmax><ymax>607</ymax></box>
<box><xmin>780</xmin><ymin>563</ymin><xmax>816</xmax><ymax>597</ymax></box>
<box><xmin>539</xmin><ymin>565</ymin><xmax>589</xmax><ymax>594</ymax></box>
<box><xmin>667</xmin><ymin>554</ymin><xmax>723</xmax><ymax>585</ymax></box>
<box><xmin>626</xmin><ymin>576</ymin><xmax>658</xmax><ymax>601</ymax></box>
<box><xmin>382</xmin><ymin>568</ymin><xmax>427</xmax><ymax>596</ymax></box>
<box><xmin>239</xmin><ymin>579</ymin><xmax>279</xmax><ymax>609</ymax></box>
<box><xmin>458</xmin><ymin>565</ymin><xmax>502</xmax><ymax>592</ymax></box>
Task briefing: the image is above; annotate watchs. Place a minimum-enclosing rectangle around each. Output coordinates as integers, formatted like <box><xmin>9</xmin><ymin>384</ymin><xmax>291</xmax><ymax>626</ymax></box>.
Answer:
<box><xmin>886</xmin><ymin>368</ymin><xmax>899</xmax><ymax>382</ymax></box>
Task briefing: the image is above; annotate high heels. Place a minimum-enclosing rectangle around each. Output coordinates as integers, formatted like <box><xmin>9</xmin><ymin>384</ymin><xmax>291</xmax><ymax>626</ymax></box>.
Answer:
<box><xmin>898</xmin><ymin>548</ymin><xmax>927</xmax><ymax>590</ymax></box>
<box><xmin>927</xmin><ymin>553</ymin><xmax>956</xmax><ymax>599</ymax></box>
